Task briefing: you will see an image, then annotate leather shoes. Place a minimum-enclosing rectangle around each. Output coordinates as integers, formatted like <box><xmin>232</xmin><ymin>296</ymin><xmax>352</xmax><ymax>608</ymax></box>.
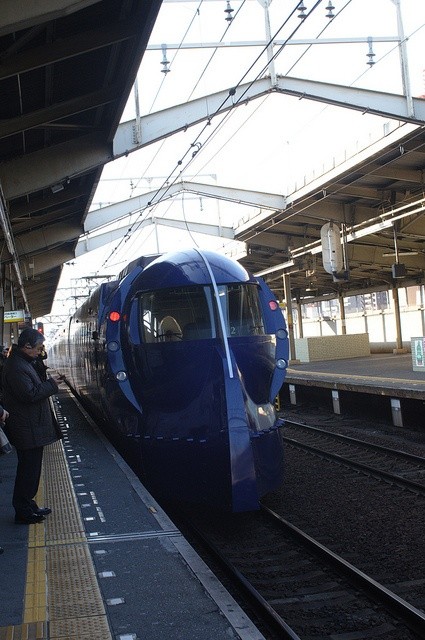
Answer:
<box><xmin>16</xmin><ymin>514</ymin><xmax>45</xmax><ymax>524</ymax></box>
<box><xmin>35</xmin><ymin>507</ymin><xmax>52</xmax><ymax>515</ymax></box>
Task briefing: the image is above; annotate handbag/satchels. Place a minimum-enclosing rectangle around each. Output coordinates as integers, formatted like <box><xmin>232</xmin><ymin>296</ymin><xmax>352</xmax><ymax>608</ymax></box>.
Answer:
<box><xmin>0</xmin><ymin>425</ymin><xmax>12</xmax><ymax>454</ymax></box>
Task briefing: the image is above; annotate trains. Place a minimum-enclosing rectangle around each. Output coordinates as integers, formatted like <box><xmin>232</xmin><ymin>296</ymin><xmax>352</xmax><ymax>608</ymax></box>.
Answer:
<box><xmin>46</xmin><ymin>247</ymin><xmax>289</xmax><ymax>512</ymax></box>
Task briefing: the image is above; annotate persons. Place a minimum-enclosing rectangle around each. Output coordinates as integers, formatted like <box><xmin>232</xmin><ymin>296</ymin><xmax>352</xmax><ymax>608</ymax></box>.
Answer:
<box><xmin>0</xmin><ymin>405</ymin><xmax>10</xmax><ymax>427</ymax></box>
<box><xmin>1</xmin><ymin>328</ymin><xmax>66</xmax><ymax>525</ymax></box>
